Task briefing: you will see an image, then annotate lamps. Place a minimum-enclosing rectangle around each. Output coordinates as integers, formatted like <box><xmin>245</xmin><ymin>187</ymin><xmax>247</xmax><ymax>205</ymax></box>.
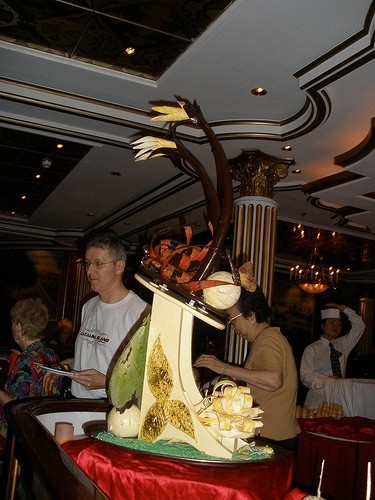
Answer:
<box><xmin>284</xmin><ymin>222</ymin><xmax>345</xmax><ymax>296</ymax></box>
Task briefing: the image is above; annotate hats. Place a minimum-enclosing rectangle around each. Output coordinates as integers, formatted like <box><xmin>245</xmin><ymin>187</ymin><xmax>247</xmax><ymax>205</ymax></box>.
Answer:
<box><xmin>320</xmin><ymin>308</ymin><xmax>340</xmax><ymax>319</ymax></box>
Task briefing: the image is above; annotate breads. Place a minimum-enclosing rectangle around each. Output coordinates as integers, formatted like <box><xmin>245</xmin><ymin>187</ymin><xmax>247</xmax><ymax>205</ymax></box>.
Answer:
<box><xmin>296</xmin><ymin>401</ymin><xmax>342</xmax><ymax>419</ymax></box>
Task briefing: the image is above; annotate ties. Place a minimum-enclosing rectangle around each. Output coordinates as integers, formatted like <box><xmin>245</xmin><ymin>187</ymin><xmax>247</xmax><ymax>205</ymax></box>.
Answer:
<box><xmin>328</xmin><ymin>343</ymin><xmax>343</xmax><ymax>378</ymax></box>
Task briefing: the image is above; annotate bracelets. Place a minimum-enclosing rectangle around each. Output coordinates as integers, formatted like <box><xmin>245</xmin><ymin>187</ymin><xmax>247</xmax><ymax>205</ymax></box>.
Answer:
<box><xmin>223</xmin><ymin>364</ymin><xmax>230</xmax><ymax>374</ymax></box>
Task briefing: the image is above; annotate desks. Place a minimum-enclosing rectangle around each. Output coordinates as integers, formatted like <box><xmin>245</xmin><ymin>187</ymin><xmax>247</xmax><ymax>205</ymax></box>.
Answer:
<box><xmin>324</xmin><ymin>376</ymin><xmax>375</xmax><ymax>419</ymax></box>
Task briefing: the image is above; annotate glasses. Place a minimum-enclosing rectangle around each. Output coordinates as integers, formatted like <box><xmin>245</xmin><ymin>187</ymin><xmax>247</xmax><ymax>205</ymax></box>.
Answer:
<box><xmin>81</xmin><ymin>258</ymin><xmax>120</xmax><ymax>269</ymax></box>
<box><xmin>227</xmin><ymin>312</ymin><xmax>244</xmax><ymax>327</ymax></box>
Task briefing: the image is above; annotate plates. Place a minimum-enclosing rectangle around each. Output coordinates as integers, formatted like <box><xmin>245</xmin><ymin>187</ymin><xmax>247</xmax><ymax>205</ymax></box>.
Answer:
<box><xmin>38</xmin><ymin>363</ymin><xmax>78</xmax><ymax>376</ymax></box>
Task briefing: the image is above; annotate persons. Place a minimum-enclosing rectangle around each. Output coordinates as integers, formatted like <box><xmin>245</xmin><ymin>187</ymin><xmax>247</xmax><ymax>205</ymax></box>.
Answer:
<box><xmin>192</xmin><ymin>283</ymin><xmax>301</xmax><ymax>458</ymax></box>
<box><xmin>300</xmin><ymin>304</ymin><xmax>365</xmax><ymax>409</ymax></box>
<box><xmin>0</xmin><ymin>228</ymin><xmax>152</xmax><ymax>471</ymax></box>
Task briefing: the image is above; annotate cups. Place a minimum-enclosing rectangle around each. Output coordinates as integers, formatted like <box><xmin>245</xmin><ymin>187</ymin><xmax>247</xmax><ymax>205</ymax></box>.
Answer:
<box><xmin>55</xmin><ymin>423</ymin><xmax>75</xmax><ymax>445</ymax></box>
